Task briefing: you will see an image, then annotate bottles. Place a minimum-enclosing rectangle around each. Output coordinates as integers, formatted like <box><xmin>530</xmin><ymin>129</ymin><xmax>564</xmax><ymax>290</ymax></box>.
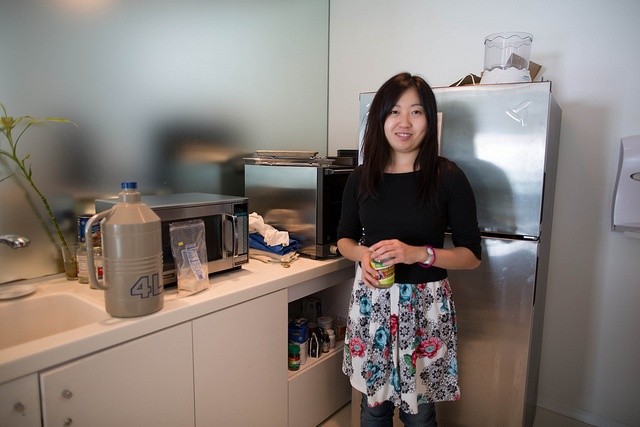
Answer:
<box><xmin>327</xmin><ymin>330</ymin><xmax>335</xmax><ymax>348</ymax></box>
<box><xmin>322</xmin><ymin>334</ymin><xmax>330</xmax><ymax>353</ymax></box>
<box><xmin>289</xmin><ymin>322</ymin><xmax>308</xmax><ymax>365</ymax></box>
<box><xmin>317</xmin><ymin>315</ymin><xmax>333</xmax><ymax>330</ymax></box>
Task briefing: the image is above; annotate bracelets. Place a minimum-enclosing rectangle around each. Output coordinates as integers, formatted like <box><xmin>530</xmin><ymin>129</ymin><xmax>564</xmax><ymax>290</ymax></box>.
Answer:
<box><xmin>418</xmin><ymin>245</ymin><xmax>436</xmax><ymax>268</ymax></box>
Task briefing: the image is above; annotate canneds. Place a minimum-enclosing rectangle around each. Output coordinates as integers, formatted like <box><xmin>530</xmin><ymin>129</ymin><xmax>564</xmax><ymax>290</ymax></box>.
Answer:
<box><xmin>331</xmin><ymin>316</ymin><xmax>345</xmax><ymax>343</ymax></box>
<box><xmin>89</xmin><ymin>246</ymin><xmax>104</xmax><ymax>289</ymax></box>
<box><xmin>76</xmin><ymin>243</ymin><xmax>89</xmax><ymax>283</ymax></box>
<box><xmin>318</xmin><ymin>316</ymin><xmax>332</xmax><ymax>332</ymax></box>
<box><xmin>76</xmin><ymin>216</ymin><xmax>101</xmax><ymax>246</ymax></box>
<box><xmin>370</xmin><ymin>248</ymin><xmax>396</xmax><ymax>290</ymax></box>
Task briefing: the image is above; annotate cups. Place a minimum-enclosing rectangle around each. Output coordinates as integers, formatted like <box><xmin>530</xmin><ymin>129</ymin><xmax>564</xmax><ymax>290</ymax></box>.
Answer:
<box><xmin>333</xmin><ymin>315</ymin><xmax>346</xmax><ymax>341</ymax></box>
<box><xmin>61</xmin><ymin>243</ymin><xmax>77</xmax><ymax>280</ymax></box>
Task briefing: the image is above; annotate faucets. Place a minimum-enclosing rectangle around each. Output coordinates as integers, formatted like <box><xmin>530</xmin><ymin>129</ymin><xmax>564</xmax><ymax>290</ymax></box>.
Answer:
<box><xmin>1</xmin><ymin>232</ymin><xmax>31</xmax><ymax>250</ymax></box>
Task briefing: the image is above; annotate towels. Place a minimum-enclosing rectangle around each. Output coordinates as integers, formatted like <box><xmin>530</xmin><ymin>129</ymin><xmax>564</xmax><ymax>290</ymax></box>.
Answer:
<box><xmin>248</xmin><ymin>213</ymin><xmax>289</xmax><ymax>247</ymax></box>
<box><xmin>248</xmin><ymin>233</ymin><xmax>298</xmax><ymax>255</ymax></box>
<box><xmin>249</xmin><ymin>248</ymin><xmax>299</xmax><ymax>263</ymax></box>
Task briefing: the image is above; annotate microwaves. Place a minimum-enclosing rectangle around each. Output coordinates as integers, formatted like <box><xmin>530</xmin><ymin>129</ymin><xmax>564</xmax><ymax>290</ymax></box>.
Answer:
<box><xmin>94</xmin><ymin>192</ymin><xmax>250</xmax><ymax>285</ymax></box>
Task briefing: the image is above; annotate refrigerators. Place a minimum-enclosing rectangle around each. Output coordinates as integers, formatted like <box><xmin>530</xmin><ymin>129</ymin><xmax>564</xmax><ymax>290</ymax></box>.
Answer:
<box><xmin>350</xmin><ymin>81</ymin><xmax>562</xmax><ymax>427</ymax></box>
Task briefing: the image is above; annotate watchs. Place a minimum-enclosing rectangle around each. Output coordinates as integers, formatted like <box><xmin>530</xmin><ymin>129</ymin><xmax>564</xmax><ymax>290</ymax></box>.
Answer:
<box><xmin>423</xmin><ymin>248</ymin><xmax>435</xmax><ymax>264</ymax></box>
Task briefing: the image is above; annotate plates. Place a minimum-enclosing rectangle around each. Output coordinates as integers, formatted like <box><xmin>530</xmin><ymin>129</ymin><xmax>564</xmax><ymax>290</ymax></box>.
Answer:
<box><xmin>253</xmin><ymin>150</ymin><xmax>319</xmax><ymax>159</ymax></box>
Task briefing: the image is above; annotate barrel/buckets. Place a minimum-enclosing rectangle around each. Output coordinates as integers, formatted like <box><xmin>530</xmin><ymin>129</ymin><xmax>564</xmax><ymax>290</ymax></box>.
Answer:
<box><xmin>84</xmin><ymin>180</ymin><xmax>165</xmax><ymax>320</ymax></box>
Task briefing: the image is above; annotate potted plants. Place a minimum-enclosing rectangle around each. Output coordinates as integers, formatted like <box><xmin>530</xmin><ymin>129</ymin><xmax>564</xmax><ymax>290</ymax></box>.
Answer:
<box><xmin>1</xmin><ymin>102</ymin><xmax>82</xmax><ymax>280</ymax></box>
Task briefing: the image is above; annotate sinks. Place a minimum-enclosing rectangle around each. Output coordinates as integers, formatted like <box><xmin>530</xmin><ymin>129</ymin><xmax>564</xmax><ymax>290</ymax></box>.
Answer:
<box><xmin>0</xmin><ymin>291</ymin><xmax>109</xmax><ymax>351</ymax></box>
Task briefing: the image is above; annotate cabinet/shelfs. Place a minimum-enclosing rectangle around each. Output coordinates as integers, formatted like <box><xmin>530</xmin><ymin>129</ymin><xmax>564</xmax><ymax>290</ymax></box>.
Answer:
<box><xmin>192</xmin><ymin>277</ymin><xmax>288</xmax><ymax>426</ymax></box>
<box><xmin>288</xmin><ymin>258</ymin><xmax>355</xmax><ymax>427</ymax></box>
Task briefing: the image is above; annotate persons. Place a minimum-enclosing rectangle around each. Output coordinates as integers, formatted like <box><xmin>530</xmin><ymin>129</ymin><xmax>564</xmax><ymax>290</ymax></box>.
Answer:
<box><xmin>335</xmin><ymin>72</ymin><xmax>483</xmax><ymax>427</ymax></box>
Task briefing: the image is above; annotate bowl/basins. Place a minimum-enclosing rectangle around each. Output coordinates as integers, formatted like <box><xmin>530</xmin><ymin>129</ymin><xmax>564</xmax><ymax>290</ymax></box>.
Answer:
<box><xmin>477</xmin><ymin>29</ymin><xmax>534</xmax><ymax>85</ymax></box>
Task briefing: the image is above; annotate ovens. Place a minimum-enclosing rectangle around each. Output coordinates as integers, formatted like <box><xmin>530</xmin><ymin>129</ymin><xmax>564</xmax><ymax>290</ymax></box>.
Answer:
<box><xmin>245</xmin><ymin>163</ymin><xmax>359</xmax><ymax>259</ymax></box>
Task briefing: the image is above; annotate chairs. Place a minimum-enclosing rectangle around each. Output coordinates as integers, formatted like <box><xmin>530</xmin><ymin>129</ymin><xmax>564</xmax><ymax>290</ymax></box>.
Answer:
<box><xmin>0</xmin><ymin>305</ymin><xmax>195</xmax><ymax>426</ymax></box>
